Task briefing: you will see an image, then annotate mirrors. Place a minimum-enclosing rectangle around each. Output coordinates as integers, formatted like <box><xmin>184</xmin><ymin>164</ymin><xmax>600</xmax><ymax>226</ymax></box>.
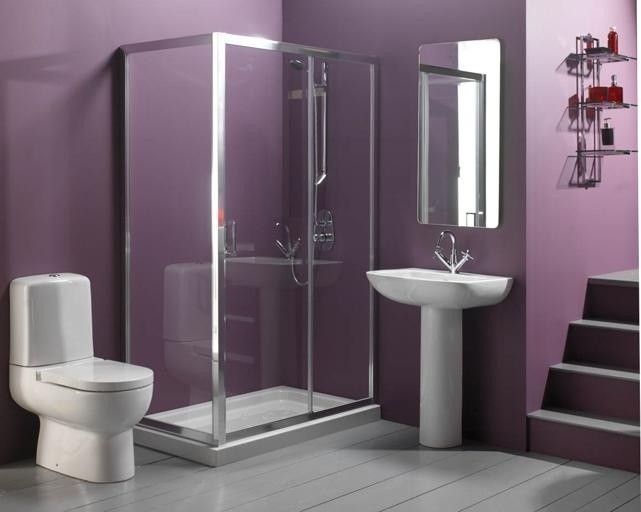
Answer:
<box><xmin>417</xmin><ymin>38</ymin><xmax>501</xmax><ymax>229</ymax></box>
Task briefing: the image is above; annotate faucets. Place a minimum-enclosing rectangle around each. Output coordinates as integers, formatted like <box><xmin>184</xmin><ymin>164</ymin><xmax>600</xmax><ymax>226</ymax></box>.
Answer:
<box><xmin>273</xmin><ymin>220</ymin><xmax>303</xmax><ymax>257</ymax></box>
<box><xmin>431</xmin><ymin>228</ymin><xmax>473</xmax><ymax>272</ymax></box>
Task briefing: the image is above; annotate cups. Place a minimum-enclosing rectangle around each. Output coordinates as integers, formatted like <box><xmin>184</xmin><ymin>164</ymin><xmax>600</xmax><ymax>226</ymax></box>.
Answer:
<box><xmin>589</xmin><ymin>86</ymin><xmax>608</xmax><ymax>102</ymax></box>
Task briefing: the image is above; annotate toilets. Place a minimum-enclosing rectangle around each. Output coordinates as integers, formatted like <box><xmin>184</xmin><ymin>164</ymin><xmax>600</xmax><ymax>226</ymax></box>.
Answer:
<box><xmin>7</xmin><ymin>272</ymin><xmax>154</xmax><ymax>484</ymax></box>
<box><xmin>162</xmin><ymin>260</ymin><xmax>212</xmax><ymax>404</ymax></box>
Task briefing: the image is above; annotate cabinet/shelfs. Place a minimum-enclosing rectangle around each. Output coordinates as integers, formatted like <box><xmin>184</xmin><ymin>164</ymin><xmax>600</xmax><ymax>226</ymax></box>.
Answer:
<box><xmin>565</xmin><ymin>34</ymin><xmax>637</xmax><ymax>190</ymax></box>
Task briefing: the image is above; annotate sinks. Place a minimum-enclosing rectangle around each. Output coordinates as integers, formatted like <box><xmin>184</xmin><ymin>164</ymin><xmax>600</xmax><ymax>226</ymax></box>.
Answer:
<box><xmin>366</xmin><ymin>267</ymin><xmax>514</xmax><ymax>308</ymax></box>
<box><xmin>226</xmin><ymin>255</ymin><xmax>344</xmax><ymax>287</ymax></box>
